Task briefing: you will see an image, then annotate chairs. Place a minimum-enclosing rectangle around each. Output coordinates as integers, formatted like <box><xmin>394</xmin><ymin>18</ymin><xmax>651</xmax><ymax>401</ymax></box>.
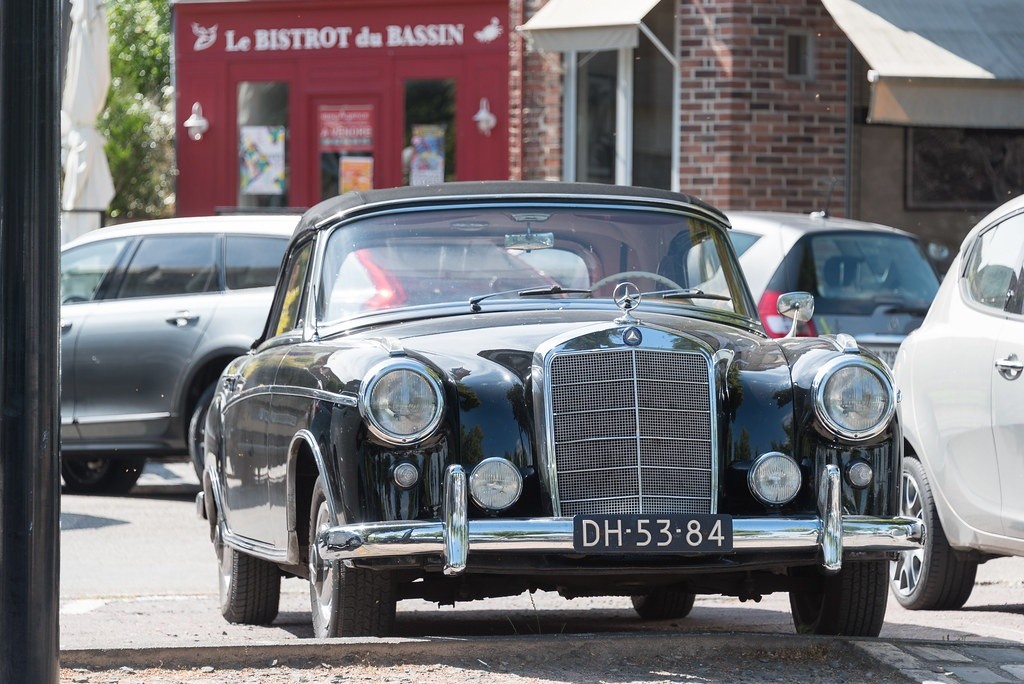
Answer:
<box><xmin>823</xmin><ymin>257</ymin><xmax>863</xmax><ymax>291</ymax></box>
<box><xmin>868</xmin><ymin>257</ymin><xmax>901</xmax><ymax>291</ymax></box>
<box><xmin>144</xmin><ymin>272</ymin><xmax>184</xmax><ymax>296</ymax></box>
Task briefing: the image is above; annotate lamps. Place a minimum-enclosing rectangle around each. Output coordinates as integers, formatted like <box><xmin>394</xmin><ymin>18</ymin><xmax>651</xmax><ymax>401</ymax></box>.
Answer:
<box><xmin>184</xmin><ymin>101</ymin><xmax>210</xmax><ymax>141</ymax></box>
<box><xmin>470</xmin><ymin>96</ymin><xmax>497</xmax><ymax>137</ymax></box>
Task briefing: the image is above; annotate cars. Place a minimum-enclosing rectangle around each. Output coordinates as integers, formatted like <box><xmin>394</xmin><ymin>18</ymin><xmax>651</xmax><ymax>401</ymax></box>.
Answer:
<box><xmin>198</xmin><ymin>179</ymin><xmax>928</xmax><ymax>639</ymax></box>
<box><xmin>510</xmin><ymin>207</ymin><xmax>941</xmax><ymax>370</ymax></box>
<box><xmin>886</xmin><ymin>191</ymin><xmax>1024</xmax><ymax>610</ymax></box>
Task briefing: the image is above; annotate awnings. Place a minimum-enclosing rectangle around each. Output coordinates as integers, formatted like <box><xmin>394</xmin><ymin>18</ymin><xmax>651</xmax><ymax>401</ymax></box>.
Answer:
<box><xmin>515</xmin><ymin>0</ymin><xmax>661</xmax><ymax>52</ymax></box>
<box><xmin>821</xmin><ymin>0</ymin><xmax>1024</xmax><ymax>130</ymax></box>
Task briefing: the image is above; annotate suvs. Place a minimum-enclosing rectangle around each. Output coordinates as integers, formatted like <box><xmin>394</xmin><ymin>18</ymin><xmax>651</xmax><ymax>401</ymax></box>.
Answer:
<box><xmin>58</xmin><ymin>208</ymin><xmax>570</xmax><ymax>497</ymax></box>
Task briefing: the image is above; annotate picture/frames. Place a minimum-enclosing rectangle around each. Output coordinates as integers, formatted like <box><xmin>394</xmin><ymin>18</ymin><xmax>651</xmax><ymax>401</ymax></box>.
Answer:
<box><xmin>902</xmin><ymin>125</ymin><xmax>1024</xmax><ymax>211</ymax></box>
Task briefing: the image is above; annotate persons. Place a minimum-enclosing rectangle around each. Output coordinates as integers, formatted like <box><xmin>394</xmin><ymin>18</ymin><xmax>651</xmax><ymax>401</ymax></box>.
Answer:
<box><xmin>846</xmin><ymin>236</ymin><xmax>915</xmax><ymax>299</ymax></box>
